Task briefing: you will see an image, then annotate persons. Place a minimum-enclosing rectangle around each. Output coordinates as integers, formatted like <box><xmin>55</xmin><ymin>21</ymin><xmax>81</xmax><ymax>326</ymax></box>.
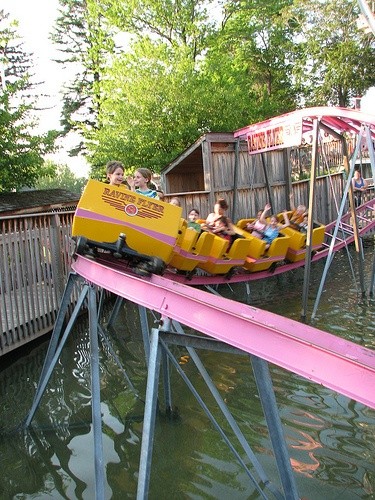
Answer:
<box><xmin>246</xmin><ymin>209</ymin><xmax>266</xmax><ymax>239</ymax></box>
<box><xmin>155</xmin><ymin>190</ymin><xmax>165</xmax><ymax>202</ymax></box>
<box><xmin>298</xmin><ymin>214</ymin><xmax>312</xmax><ymax>234</ymax></box>
<box><xmin>126</xmin><ymin>168</ymin><xmax>159</xmax><ymax>201</ymax></box>
<box><xmin>290</xmin><ymin>193</ymin><xmax>308</xmax><ymax>231</ymax></box>
<box><xmin>187</xmin><ymin>209</ymin><xmax>201</xmax><ymax>234</ymax></box>
<box><xmin>105</xmin><ymin>161</ymin><xmax>128</xmax><ymax>189</ymax></box>
<box><xmin>206</xmin><ymin>217</ymin><xmax>231</xmax><ymax>254</ymax></box>
<box><xmin>352</xmin><ymin>170</ymin><xmax>366</xmax><ymax>207</ymax></box>
<box><xmin>170</xmin><ymin>197</ymin><xmax>181</xmax><ymax>207</ymax></box>
<box><xmin>201</xmin><ymin>198</ymin><xmax>236</xmax><ymax>236</ymax></box>
<box><xmin>260</xmin><ymin>202</ymin><xmax>290</xmax><ymax>250</ymax></box>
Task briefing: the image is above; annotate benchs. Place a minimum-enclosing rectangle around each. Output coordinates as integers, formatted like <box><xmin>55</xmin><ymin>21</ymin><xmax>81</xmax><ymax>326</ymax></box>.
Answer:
<box><xmin>170</xmin><ymin>210</ymin><xmax>327</xmax><ymax>275</ymax></box>
